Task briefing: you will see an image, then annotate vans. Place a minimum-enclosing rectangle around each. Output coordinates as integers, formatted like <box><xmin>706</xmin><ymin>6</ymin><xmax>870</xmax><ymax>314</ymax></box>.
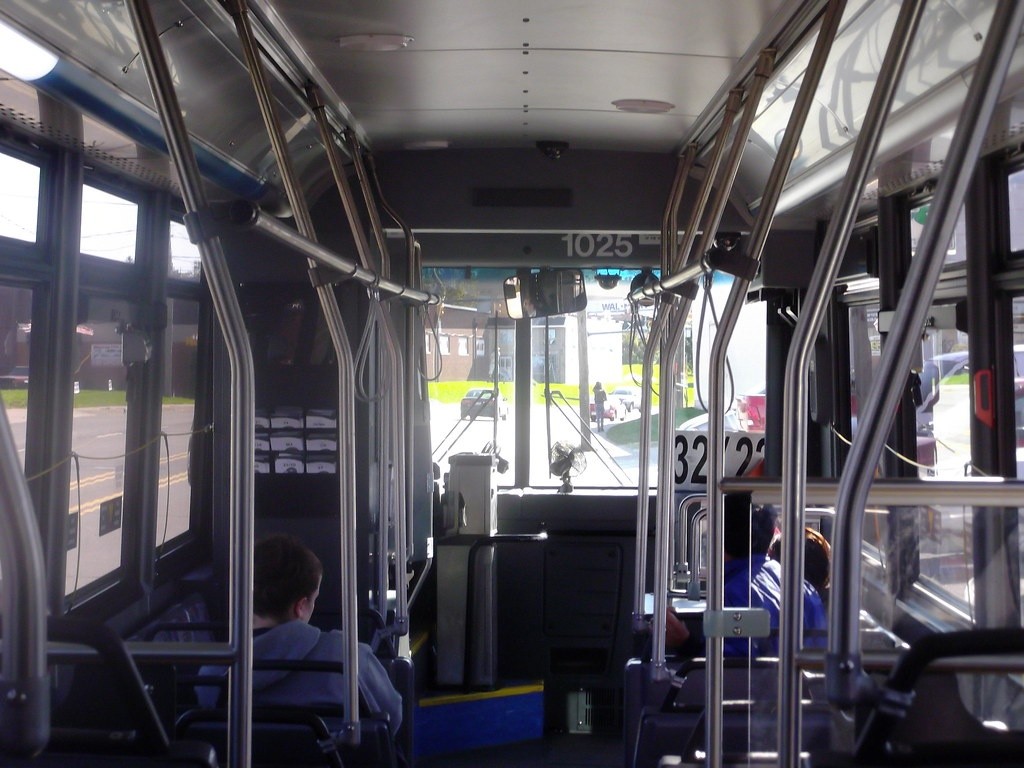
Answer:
<box><xmin>612</xmin><ymin>386</ymin><xmax>641</xmax><ymax>413</ymax></box>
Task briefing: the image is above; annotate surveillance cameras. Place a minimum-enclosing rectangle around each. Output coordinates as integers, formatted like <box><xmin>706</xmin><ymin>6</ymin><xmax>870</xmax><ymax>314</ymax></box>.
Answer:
<box><xmin>713</xmin><ymin>232</ymin><xmax>742</xmax><ymax>250</ymax></box>
<box><xmin>536</xmin><ymin>141</ymin><xmax>569</xmax><ymax>160</ymax></box>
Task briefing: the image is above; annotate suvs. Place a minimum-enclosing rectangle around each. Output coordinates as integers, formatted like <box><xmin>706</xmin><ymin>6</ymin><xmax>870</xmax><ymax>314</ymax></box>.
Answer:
<box><xmin>919</xmin><ymin>344</ymin><xmax>1024</xmax><ymax>446</ymax></box>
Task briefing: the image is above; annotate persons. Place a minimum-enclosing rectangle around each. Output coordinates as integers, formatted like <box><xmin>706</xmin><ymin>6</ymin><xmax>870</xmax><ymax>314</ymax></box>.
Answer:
<box><xmin>521</xmin><ymin>296</ymin><xmax>546</xmax><ymax>319</ymax></box>
<box><xmin>593</xmin><ymin>382</ymin><xmax>607</xmax><ymax>432</ymax></box>
<box><xmin>488</xmin><ymin>346</ymin><xmax>501</xmax><ymax>382</ymax></box>
<box><xmin>649</xmin><ymin>502</ymin><xmax>830</xmax><ymax>666</ymax></box>
<box><xmin>195</xmin><ymin>535</ymin><xmax>405</xmax><ymax>737</ymax></box>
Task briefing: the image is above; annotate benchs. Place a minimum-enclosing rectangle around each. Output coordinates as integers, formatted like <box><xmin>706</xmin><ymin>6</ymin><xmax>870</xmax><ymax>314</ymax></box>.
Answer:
<box><xmin>1</xmin><ymin>623</ymin><xmax>415</xmax><ymax>768</ymax></box>
<box><xmin>635</xmin><ymin>626</ymin><xmax>1024</xmax><ymax>768</ymax></box>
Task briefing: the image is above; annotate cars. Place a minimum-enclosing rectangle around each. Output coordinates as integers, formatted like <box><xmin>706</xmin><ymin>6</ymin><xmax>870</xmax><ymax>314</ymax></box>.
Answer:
<box><xmin>460</xmin><ymin>388</ymin><xmax>509</xmax><ymax>421</ymax></box>
<box><xmin>590</xmin><ymin>394</ymin><xmax>627</xmax><ymax>421</ymax></box>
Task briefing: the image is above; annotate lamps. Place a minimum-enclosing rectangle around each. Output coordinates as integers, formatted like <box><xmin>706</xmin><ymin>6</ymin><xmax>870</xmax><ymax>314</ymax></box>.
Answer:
<box><xmin>0</xmin><ymin>11</ymin><xmax>273</xmax><ymax>201</ymax></box>
<box><xmin>747</xmin><ymin>34</ymin><xmax>1024</xmax><ymax>220</ymax></box>
